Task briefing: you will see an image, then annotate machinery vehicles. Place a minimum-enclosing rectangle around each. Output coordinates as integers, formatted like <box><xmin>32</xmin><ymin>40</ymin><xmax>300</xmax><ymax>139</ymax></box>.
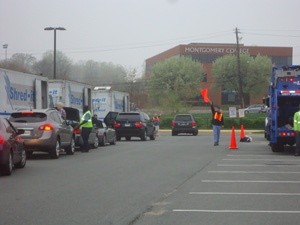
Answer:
<box><xmin>265</xmin><ymin>65</ymin><xmax>300</xmax><ymax>152</ymax></box>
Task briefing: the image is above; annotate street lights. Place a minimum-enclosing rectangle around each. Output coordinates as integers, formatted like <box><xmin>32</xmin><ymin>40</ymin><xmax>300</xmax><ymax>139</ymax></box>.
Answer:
<box><xmin>44</xmin><ymin>27</ymin><xmax>66</xmax><ymax>80</ymax></box>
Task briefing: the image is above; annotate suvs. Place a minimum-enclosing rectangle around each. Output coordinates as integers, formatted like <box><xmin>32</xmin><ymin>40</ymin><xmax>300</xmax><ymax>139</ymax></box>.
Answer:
<box><xmin>9</xmin><ymin>108</ymin><xmax>76</xmax><ymax>158</ymax></box>
<box><xmin>103</xmin><ymin>112</ymin><xmax>156</xmax><ymax>141</ymax></box>
<box><xmin>171</xmin><ymin>114</ymin><xmax>198</xmax><ymax>136</ymax></box>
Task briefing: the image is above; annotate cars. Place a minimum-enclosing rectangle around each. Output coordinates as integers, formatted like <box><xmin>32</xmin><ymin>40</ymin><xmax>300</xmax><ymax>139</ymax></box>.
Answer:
<box><xmin>98</xmin><ymin>121</ymin><xmax>117</xmax><ymax>145</ymax></box>
<box><xmin>54</xmin><ymin>107</ymin><xmax>100</xmax><ymax>148</ymax></box>
<box><xmin>0</xmin><ymin>118</ymin><xmax>27</xmax><ymax>175</ymax></box>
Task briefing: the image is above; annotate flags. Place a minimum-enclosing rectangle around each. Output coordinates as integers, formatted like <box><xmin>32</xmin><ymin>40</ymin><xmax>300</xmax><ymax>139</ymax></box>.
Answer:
<box><xmin>201</xmin><ymin>87</ymin><xmax>211</xmax><ymax>102</ymax></box>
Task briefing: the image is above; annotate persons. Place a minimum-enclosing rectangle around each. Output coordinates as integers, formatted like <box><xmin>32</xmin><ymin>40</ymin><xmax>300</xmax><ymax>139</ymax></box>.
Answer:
<box><xmin>73</xmin><ymin>105</ymin><xmax>93</xmax><ymax>152</ymax></box>
<box><xmin>293</xmin><ymin>103</ymin><xmax>300</xmax><ymax>156</ymax></box>
<box><xmin>209</xmin><ymin>100</ymin><xmax>225</xmax><ymax>146</ymax></box>
<box><xmin>150</xmin><ymin>113</ymin><xmax>162</xmax><ymax>135</ymax></box>
<box><xmin>56</xmin><ymin>102</ymin><xmax>66</xmax><ymax>120</ymax></box>
